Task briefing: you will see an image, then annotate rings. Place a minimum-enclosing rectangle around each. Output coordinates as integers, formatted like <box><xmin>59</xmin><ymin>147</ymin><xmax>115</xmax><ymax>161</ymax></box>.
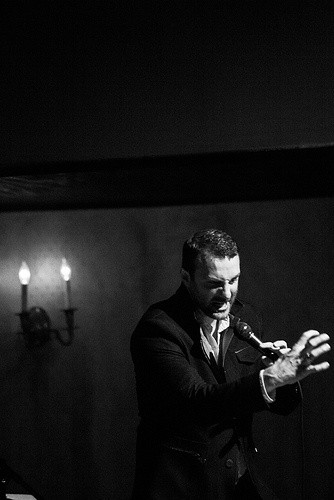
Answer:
<box><xmin>305</xmin><ymin>352</ymin><xmax>313</xmax><ymax>364</ymax></box>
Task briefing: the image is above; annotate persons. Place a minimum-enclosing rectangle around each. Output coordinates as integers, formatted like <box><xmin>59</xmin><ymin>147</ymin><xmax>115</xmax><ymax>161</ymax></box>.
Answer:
<box><xmin>129</xmin><ymin>228</ymin><xmax>331</xmax><ymax>500</ymax></box>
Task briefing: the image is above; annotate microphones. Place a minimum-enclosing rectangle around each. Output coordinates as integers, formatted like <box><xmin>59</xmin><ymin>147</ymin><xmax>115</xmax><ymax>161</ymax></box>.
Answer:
<box><xmin>236</xmin><ymin>322</ymin><xmax>279</xmax><ymax>362</ymax></box>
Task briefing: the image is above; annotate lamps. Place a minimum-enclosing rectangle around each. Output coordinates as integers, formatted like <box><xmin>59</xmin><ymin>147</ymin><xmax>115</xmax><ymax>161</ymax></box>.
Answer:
<box><xmin>14</xmin><ymin>257</ymin><xmax>77</xmax><ymax>352</ymax></box>
